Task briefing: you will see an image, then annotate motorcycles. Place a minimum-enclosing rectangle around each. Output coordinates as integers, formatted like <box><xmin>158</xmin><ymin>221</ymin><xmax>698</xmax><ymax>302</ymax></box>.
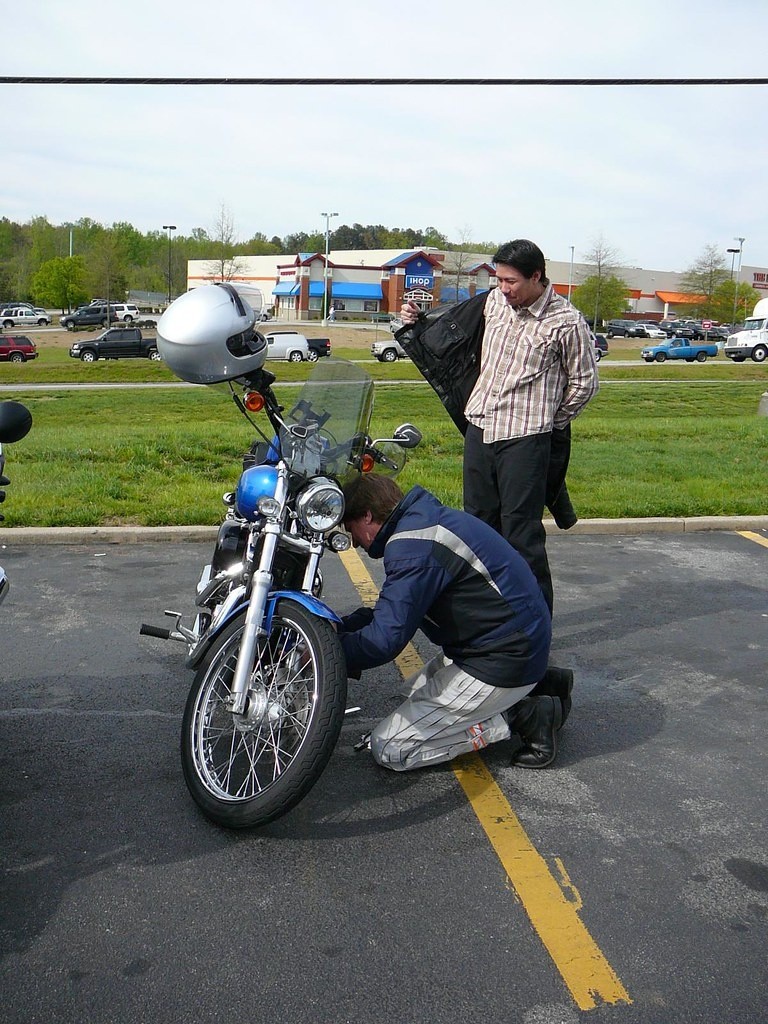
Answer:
<box><xmin>139</xmin><ymin>355</ymin><xmax>423</xmax><ymax>832</ymax></box>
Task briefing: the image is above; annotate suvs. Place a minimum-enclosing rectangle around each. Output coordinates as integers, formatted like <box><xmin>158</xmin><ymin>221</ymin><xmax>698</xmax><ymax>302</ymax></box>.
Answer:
<box><xmin>0</xmin><ymin>334</ymin><xmax>39</xmax><ymax>362</ymax></box>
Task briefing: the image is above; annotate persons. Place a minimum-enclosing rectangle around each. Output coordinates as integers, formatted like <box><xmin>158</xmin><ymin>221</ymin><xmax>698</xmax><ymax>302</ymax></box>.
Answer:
<box><xmin>329</xmin><ymin>304</ymin><xmax>336</xmax><ymax>322</ymax></box>
<box><xmin>264</xmin><ymin>474</ymin><xmax>574</xmax><ymax>772</ymax></box>
<box><xmin>399</xmin><ymin>239</ymin><xmax>599</xmax><ymax>623</ymax></box>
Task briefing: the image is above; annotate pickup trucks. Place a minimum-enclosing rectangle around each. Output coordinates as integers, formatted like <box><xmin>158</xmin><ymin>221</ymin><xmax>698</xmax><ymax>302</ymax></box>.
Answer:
<box><xmin>267</xmin><ymin>332</ymin><xmax>332</xmax><ymax>362</ymax></box>
<box><xmin>69</xmin><ymin>328</ymin><xmax>162</xmax><ymax>362</ymax></box>
<box><xmin>640</xmin><ymin>338</ymin><xmax>719</xmax><ymax>362</ymax></box>
<box><xmin>0</xmin><ymin>310</ymin><xmax>52</xmax><ymax>328</ymax></box>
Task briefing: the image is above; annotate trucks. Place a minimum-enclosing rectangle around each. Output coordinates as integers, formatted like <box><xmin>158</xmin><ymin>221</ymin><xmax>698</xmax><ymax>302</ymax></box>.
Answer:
<box><xmin>724</xmin><ymin>298</ymin><xmax>768</xmax><ymax>362</ymax></box>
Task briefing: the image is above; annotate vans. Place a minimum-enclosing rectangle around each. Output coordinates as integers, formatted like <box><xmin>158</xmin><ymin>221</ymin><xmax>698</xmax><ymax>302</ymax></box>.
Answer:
<box><xmin>263</xmin><ymin>334</ymin><xmax>309</xmax><ymax>363</ymax></box>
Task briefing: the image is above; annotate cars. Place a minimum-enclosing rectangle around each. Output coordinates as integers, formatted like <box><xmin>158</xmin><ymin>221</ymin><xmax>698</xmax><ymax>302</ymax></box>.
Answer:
<box><xmin>59</xmin><ymin>306</ymin><xmax>119</xmax><ymax>328</ymax></box>
<box><xmin>257</xmin><ymin>312</ymin><xmax>272</xmax><ymax>322</ymax></box>
<box><xmin>590</xmin><ymin>319</ymin><xmax>731</xmax><ymax>362</ymax></box>
<box><xmin>390</xmin><ymin>318</ymin><xmax>404</xmax><ymax>333</ymax></box>
<box><xmin>0</xmin><ymin>303</ymin><xmax>46</xmax><ymax>316</ymax></box>
<box><xmin>370</xmin><ymin>339</ymin><xmax>409</xmax><ymax>362</ymax></box>
<box><xmin>78</xmin><ymin>299</ymin><xmax>140</xmax><ymax>322</ymax></box>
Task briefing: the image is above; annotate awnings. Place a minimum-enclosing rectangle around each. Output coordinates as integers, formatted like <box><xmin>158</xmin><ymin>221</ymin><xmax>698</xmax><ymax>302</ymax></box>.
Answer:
<box><xmin>272</xmin><ymin>281</ymin><xmax>383</xmax><ymax>300</ymax></box>
<box><xmin>439</xmin><ymin>287</ymin><xmax>489</xmax><ymax>303</ymax></box>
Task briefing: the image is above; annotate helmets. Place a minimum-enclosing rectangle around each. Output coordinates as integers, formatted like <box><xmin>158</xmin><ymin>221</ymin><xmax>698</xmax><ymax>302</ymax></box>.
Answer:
<box><xmin>156</xmin><ymin>282</ymin><xmax>268</xmax><ymax>385</ymax></box>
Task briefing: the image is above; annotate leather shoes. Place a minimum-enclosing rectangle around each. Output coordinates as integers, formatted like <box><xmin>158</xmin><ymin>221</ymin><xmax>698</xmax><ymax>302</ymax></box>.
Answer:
<box><xmin>507</xmin><ymin>695</ymin><xmax>562</xmax><ymax>768</ymax></box>
<box><xmin>529</xmin><ymin>666</ymin><xmax>573</xmax><ymax>731</ymax></box>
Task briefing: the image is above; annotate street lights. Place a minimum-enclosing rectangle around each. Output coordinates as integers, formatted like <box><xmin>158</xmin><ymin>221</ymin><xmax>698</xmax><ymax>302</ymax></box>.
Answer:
<box><xmin>163</xmin><ymin>226</ymin><xmax>176</xmax><ymax>302</ymax></box>
<box><xmin>732</xmin><ymin>237</ymin><xmax>745</xmax><ymax>334</ymax></box>
<box><xmin>568</xmin><ymin>246</ymin><xmax>574</xmax><ymax>303</ymax></box>
<box><xmin>322</xmin><ymin>213</ymin><xmax>339</xmax><ymax>320</ymax></box>
<box><xmin>727</xmin><ymin>249</ymin><xmax>742</xmax><ymax>280</ymax></box>
